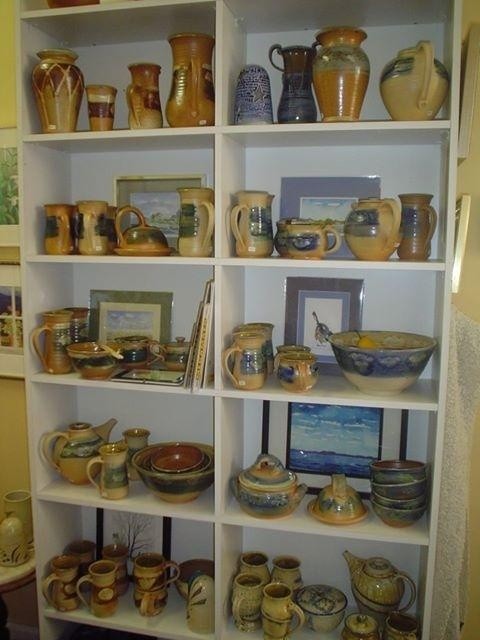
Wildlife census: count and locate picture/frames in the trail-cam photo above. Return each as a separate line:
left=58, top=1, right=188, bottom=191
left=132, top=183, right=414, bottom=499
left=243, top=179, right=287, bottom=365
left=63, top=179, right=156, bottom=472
left=457, top=19, right=480, bottom=166
left=89, top=289, right=174, bottom=347
left=449, top=194, right=472, bottom=295
left=284, top=276, right=363, bottom=377
left=281, top=178, right=381, bottom=261
left=96, top=508, right=172, bottom=582
left=262, top=399, right=408, bottom=502
left=0, top=128, right=21, bottom=250
left=0, top=263, right=25, bottom=382
left=111, top=174, right=205, bottom=250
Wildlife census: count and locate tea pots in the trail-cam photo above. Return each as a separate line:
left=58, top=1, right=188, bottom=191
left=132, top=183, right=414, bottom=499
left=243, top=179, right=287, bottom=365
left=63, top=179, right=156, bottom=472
left=39, top=416, right=118, bottom=484
left=342, top=550, right=420, bottom=622
left=229, top=451, right=310, bottom=521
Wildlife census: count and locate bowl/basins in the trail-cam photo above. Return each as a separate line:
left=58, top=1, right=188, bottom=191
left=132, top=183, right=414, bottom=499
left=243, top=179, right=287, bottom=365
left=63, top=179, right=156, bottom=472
left=326, top=329, right=438, bottom=396
left=65, top=341, right=124, bottom=381
left=131, top=440, right=215, bottom=504
left=369, top=458, right=429, bottom=528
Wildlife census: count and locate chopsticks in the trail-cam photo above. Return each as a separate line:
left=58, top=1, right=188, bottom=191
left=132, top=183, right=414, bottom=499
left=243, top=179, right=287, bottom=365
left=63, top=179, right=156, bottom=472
left=96, top=341, right=124, bottom=360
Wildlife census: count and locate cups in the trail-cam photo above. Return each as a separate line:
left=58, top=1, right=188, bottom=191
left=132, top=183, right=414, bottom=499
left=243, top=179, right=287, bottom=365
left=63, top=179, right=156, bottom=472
left=41, top=539, right=180, bottom=617
left=85, top=427, right=150, bottom=500
left=222, top=322, right=274, bottom=389
left=177, top=187, right=276, bottom=257
left=43, top=200, right=117, bottom=256
left=85, top=84, right=117, bottom=131
left=29, top=307, right=88, bottom=373
left=398, top=193, right=438, bottom=262
left=232, top=551, right=305, bottom=640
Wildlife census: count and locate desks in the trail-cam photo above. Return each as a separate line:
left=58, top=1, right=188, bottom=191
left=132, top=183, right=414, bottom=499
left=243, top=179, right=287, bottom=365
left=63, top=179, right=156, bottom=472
left=1, top=557, right=37, bottom=640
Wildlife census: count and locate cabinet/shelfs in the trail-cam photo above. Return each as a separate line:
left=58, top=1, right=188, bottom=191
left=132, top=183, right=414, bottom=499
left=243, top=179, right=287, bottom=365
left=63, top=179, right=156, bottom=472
left=14, top=0, right=461, bottom=639
